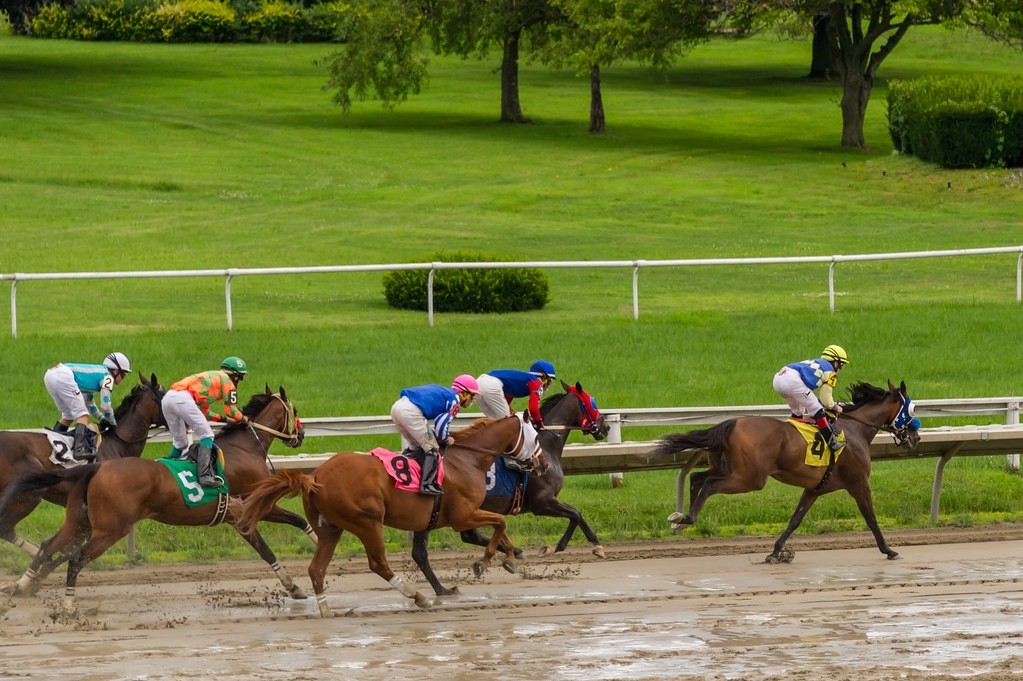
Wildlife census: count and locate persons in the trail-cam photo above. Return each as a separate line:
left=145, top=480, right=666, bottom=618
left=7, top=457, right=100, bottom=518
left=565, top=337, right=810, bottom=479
left=773, top=345, right=850, bottom=451
left=44, top=352, right=132, bottom=460
left=162, top=357, right=247, bottom=487
left=475, top=362, right=556, bottom=468
left=392, top=374, right=480, bottom=495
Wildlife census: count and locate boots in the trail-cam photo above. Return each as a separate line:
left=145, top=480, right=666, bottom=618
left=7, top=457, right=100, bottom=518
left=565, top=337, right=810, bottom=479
left=419, top=455, right=445, bottom=495
left=74, top=423, right=97, bottom=460
left=53, top=421, right=69, bottom=433
left=819, top=426, right=845, bottom=451
left=198, top=447, right=221, bottom=487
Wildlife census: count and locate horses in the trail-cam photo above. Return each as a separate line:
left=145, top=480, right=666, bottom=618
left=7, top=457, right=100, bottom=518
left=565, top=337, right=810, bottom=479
left=410, top=380, right=611, bottom=596
left=0, top=383, right=317, bottom=609
left=0, top=370, right=189, bottom=559
left=649, top=380, right=922, bottom=566
left=236, top=412, right=549, bottom=621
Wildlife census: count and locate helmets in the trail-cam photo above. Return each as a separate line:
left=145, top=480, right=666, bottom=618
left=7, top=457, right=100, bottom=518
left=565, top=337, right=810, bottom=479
left=103, top=352, right=132, bottom=372
left=821, top=345, right=849, bottom=364
left=220, top=356, right=247, bottom=375
left=529, top=360, right=557, bottom=378
left=451, top=374, right=482, bottom=395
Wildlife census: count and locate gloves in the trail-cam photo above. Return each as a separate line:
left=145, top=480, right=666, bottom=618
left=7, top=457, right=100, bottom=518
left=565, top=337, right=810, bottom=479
left=534, top=419, right=547, bottom=431
